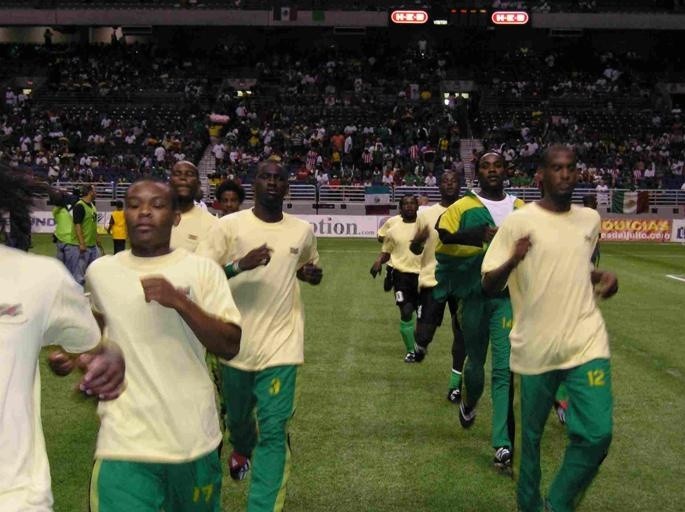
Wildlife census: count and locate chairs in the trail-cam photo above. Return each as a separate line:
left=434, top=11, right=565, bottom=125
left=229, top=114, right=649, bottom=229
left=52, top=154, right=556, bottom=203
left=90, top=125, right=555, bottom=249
left=61, top=101, right=199, bottom=131
left=310, top=86, right=388, bottom=130
left=476, top=100, right=666, bottom=150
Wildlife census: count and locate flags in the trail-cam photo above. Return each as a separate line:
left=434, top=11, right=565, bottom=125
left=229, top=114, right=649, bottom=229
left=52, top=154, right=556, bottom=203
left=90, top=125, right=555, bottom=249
left=364, top=185, right=389, bottom=217
left=612, top=192, right=649, bottom=214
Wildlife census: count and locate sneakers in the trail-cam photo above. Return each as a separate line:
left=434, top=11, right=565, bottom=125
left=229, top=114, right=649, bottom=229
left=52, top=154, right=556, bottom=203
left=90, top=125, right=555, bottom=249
left=384, top=264, right=394, bottom=291
left=448, top=388, right=461, bottom=403
left=555, top=399, right=567, bottom=424
left=229, top=452, right=249, bottom=481
left=494, top=447, right=512, bottom=468
left=415, top=343, right=426, bottom=362
left=405, top=352, right=415, bottom=363
left=459, top=401, right=476, bottom=428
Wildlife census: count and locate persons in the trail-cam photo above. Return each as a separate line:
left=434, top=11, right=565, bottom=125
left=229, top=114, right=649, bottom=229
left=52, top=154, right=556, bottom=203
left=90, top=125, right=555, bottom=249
left=166, top=161, right=246, bottom=255
left=431, top=149, right=526, bottom=472
left=371, top=170, right=464, bottom=404
left=49, top=184, right=126, bottom=284
left=47, top=179, right=242, bottom=512
left=193, top=161, right=322, bottom=511
left=1, top=28, right=685, bottom=202
left=480, top=140, right=618, bottom=511
left=0, top=163, right=128, bottom=512
